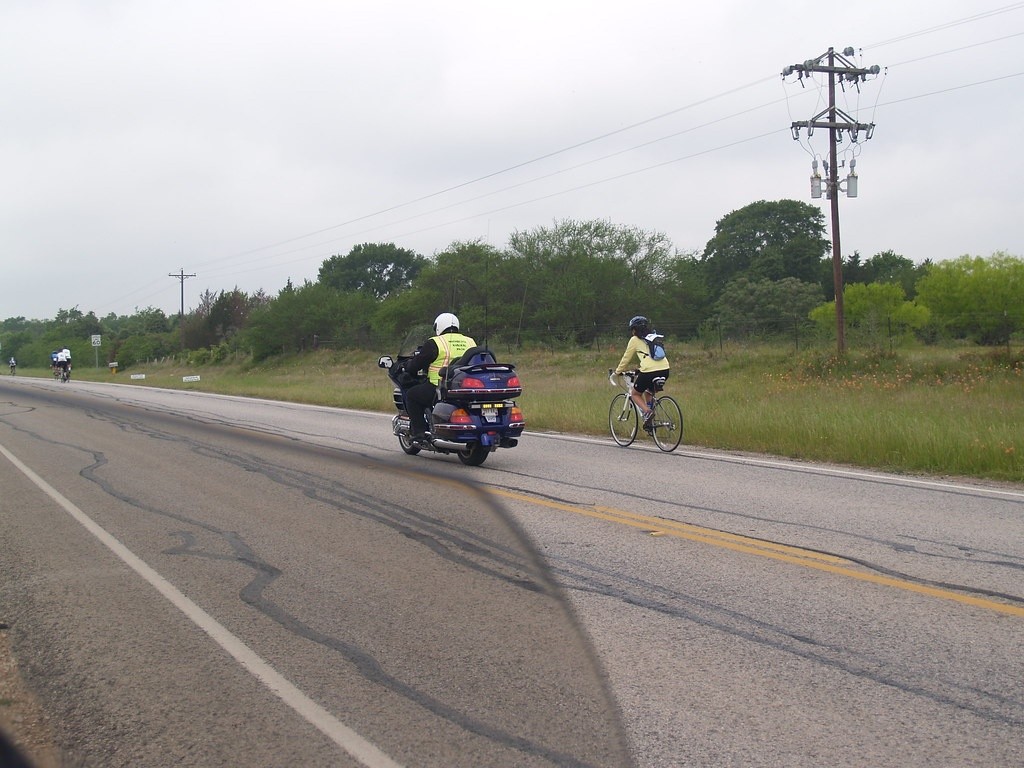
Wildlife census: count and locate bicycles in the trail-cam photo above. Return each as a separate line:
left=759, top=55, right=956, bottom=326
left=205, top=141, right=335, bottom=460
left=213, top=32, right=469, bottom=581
left=9, top=366, right=16, bottom=375
left=608, top=367, right=683, bottom=452
left=50, top=362, right=71, bottom=383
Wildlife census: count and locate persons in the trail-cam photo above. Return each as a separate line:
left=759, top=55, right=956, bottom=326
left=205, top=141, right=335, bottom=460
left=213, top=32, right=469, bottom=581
left=8, top=346, right=71, bottom=379
left=402, top=312, right=478, bottom=440
left=613, top=316, right=670, bottom=428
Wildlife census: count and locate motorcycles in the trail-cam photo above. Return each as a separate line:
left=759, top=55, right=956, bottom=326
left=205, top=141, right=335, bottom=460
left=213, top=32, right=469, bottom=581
left=378, top=343, right=526, bottom=466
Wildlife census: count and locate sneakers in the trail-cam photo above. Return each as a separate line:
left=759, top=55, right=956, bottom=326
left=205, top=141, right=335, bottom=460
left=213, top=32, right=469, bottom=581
left=642, top=410, right=654, bottom=427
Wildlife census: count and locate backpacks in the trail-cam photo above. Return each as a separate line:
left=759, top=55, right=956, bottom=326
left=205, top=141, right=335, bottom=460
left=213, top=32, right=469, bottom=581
left=644, top=333, right=665, bottom=360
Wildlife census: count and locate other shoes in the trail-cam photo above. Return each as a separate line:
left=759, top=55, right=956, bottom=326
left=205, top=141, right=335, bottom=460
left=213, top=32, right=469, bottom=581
left=409, top=432, right=425, bottom=440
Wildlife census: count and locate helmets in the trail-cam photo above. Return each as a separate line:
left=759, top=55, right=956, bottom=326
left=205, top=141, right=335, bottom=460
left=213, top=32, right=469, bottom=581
left=432, top=313, right=460, bottom=336
left=629, top=316, right=647, bottom=327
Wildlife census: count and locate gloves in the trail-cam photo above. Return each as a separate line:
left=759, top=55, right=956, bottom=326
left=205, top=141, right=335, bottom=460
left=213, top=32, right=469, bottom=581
left=615, top=372, right=624, bottom=376
left=634, top=369, right=640, bottom=375
